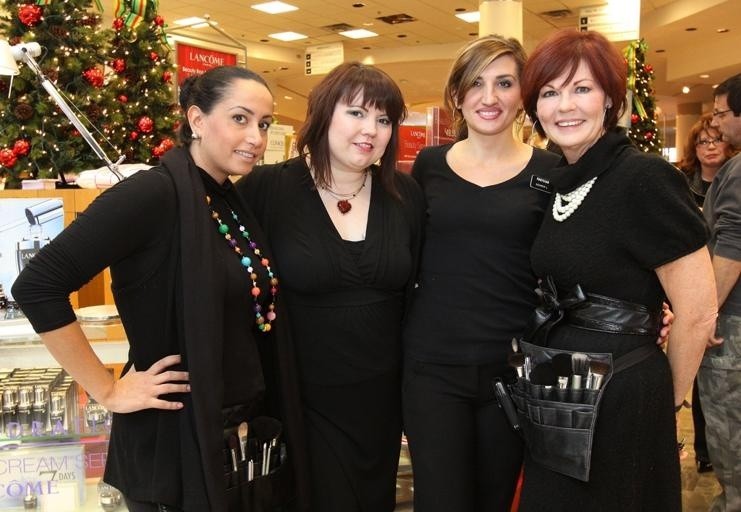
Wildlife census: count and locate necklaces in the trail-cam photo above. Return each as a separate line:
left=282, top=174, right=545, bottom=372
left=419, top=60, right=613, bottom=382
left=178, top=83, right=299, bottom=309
left=319, top=168, right=367, bottom=214
left=203, top=189, right=277, bottom=333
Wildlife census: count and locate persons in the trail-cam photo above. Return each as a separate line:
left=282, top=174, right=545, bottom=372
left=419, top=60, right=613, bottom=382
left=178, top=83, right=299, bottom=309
left=402, top=34, right=673, bottom=512
left=11, top=66, right=279, bottom=510
left=232, top=59, right=426, bottom=512
left=511, top=29, right=717, bottom=511
left=678, top=112, right=741, bottom=473
left=700, top=72, right=741, bottom=512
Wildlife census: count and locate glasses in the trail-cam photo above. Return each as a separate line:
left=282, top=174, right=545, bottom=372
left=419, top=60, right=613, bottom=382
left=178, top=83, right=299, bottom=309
left=696, top=139, right=724, bottom=147
left=712, top=110, right=733, bottom=120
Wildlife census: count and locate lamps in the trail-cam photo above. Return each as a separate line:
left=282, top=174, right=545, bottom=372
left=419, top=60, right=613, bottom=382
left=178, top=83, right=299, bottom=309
left=0, top=36, right=126, bottom=184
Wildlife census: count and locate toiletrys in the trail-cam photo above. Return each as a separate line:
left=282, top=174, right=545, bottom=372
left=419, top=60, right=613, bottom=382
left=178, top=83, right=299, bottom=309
left=0, top=363, right=125, bottom=512
